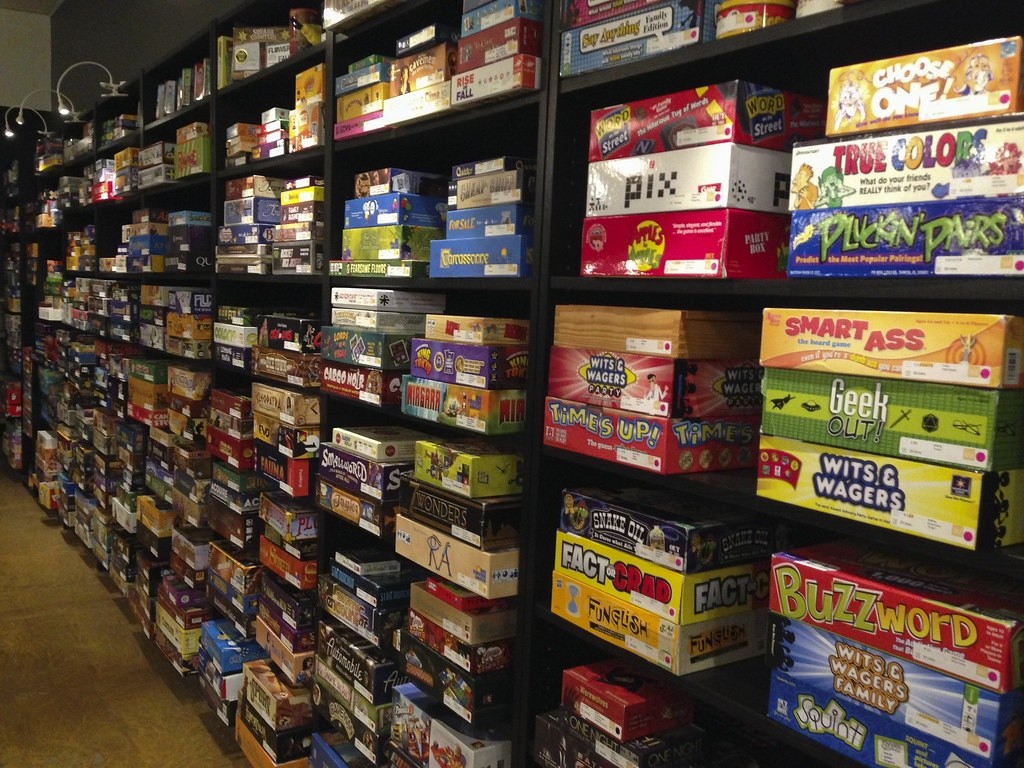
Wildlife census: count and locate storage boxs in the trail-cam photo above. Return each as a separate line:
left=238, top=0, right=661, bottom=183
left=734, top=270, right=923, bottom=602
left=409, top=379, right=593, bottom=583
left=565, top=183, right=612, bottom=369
left=0, top=0, right=1024, bottom=768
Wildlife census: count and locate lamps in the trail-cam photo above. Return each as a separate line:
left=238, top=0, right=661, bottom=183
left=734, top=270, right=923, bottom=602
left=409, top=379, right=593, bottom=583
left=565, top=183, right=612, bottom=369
left=15, top=87, right=89, bottom=125
left=56, top=61, right=129, bottom=117
left=4, top=105, right=55, bottom=139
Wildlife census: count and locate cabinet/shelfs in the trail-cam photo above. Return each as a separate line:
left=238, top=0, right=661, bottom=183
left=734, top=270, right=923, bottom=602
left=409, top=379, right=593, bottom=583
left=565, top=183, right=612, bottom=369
left=519, top=0, right=1024, bottom=768
left=0, top=0, right=555, bottom=768
